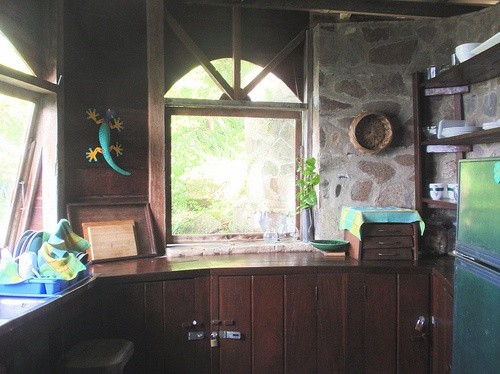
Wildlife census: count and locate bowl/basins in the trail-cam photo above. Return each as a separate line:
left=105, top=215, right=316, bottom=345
left=454, top=42, right=482, bottom=63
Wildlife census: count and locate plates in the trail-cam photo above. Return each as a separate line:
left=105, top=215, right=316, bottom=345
left=309, top=240, right=349, bottom=251
left=440, top=119, right=500, bottom=138
left=13, top=230, right=49, bottom=265
left=471, top=31, right=500, bottom=54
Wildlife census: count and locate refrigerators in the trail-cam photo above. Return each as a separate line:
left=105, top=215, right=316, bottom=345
left=450, top=156, right=500, bottom=374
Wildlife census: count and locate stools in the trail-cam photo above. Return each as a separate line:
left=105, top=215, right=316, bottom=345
left=66, top=339, right=133, bottom=374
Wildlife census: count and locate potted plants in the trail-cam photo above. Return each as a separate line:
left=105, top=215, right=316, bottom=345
left=290, top=158, right=318, bottom=242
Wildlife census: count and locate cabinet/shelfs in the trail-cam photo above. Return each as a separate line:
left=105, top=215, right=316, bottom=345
left=0, top=47, right=500, bottom=374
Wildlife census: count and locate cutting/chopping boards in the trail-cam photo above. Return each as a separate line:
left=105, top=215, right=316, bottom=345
left=82, top=220, right=138, bottom=262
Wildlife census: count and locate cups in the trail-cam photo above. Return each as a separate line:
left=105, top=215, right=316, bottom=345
left=447, top=184, right=458, bottom=199
left=429, top=183, right=444, bottom=201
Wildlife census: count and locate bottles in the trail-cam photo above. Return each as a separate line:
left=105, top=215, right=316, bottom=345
left=425, top=221, right=447, bottom=256
left=447, top=222, right=456, bottom=256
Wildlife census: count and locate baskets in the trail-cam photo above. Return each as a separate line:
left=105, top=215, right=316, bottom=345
left=348, top=111, right=393, bottom=155
